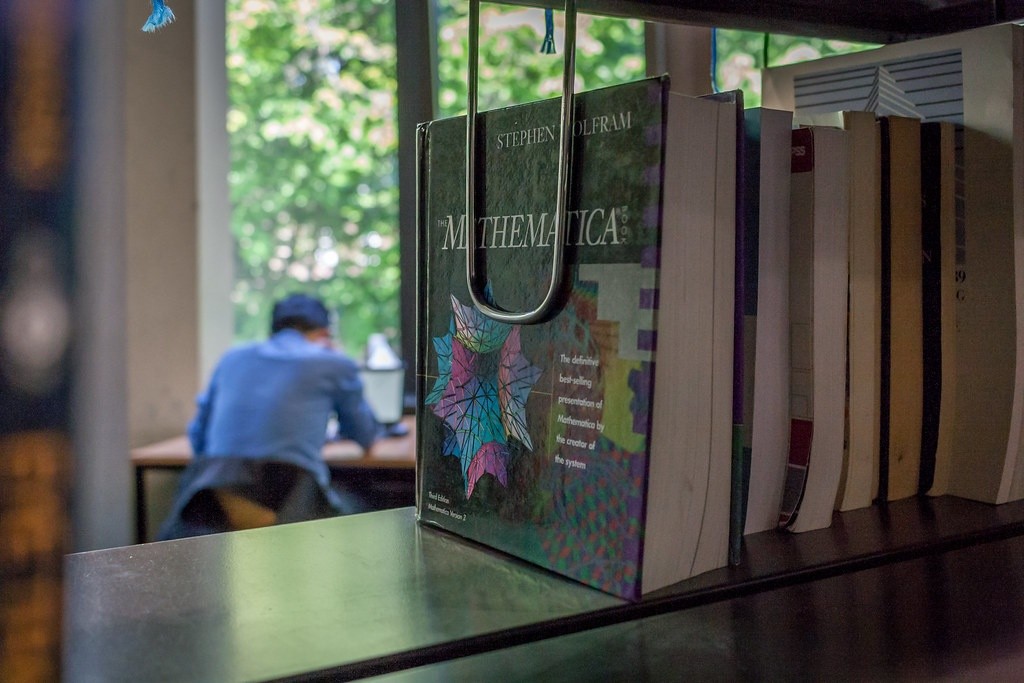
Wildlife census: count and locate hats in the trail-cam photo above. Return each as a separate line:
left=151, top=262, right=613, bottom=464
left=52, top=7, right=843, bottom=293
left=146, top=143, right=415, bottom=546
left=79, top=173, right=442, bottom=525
left=272, top=294, right=328, bottom=328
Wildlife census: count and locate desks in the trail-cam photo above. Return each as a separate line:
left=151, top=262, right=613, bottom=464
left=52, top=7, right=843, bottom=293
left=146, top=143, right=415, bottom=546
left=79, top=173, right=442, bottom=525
left=65, top=494, right=1024, bottom=683
left=132, top=414, right=416, bottom=545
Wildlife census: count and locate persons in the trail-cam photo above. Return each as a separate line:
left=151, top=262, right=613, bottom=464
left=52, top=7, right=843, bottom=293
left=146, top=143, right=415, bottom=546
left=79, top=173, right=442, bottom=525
left=189, top=294, right=380, bottom=521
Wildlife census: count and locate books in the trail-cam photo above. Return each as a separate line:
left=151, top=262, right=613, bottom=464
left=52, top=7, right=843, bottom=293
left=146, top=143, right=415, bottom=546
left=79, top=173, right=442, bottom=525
left=415, top=21, right=1023, bottom=607
